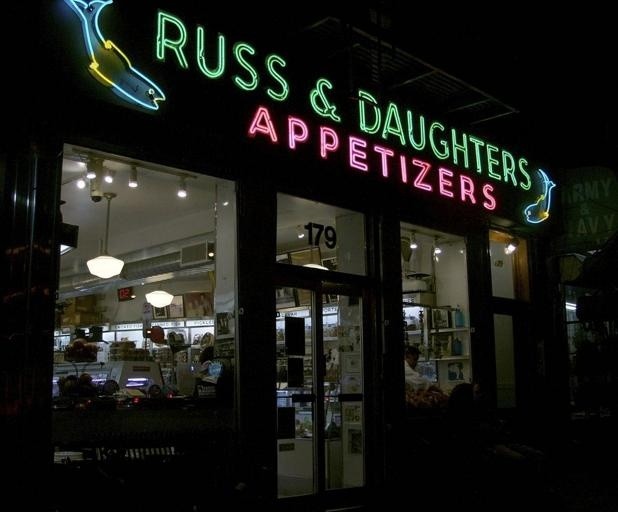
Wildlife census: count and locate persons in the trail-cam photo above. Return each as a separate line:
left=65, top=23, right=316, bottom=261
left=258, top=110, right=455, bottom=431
left=187, top=293, right=212, bottom=316
left=471, top=376, right=531, bottom=463
left=405, top=345, right=444, bottom=393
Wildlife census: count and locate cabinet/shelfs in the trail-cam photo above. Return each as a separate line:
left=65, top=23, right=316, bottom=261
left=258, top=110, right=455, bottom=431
left=277, top=326, right=470, bottom=366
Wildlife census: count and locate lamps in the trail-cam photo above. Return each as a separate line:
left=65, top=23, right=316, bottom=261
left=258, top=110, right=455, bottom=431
left=59, top=200, right=78, bottom=255
left=74, top=148, right=197, bottom=198
left=145, top=282, right=174, bottom=308
left=506, top=237, right=520, bottom=254
left=400, top=226, right=445, bottom=254
left=86, top=193, right=125, bottom=278
left=295, top=224, right=305, bottom=237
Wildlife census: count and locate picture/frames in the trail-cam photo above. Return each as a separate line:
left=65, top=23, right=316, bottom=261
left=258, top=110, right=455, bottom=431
left=152, top=305, right=168, bottom=319
left=168, top=294, right=185, bottom=318
